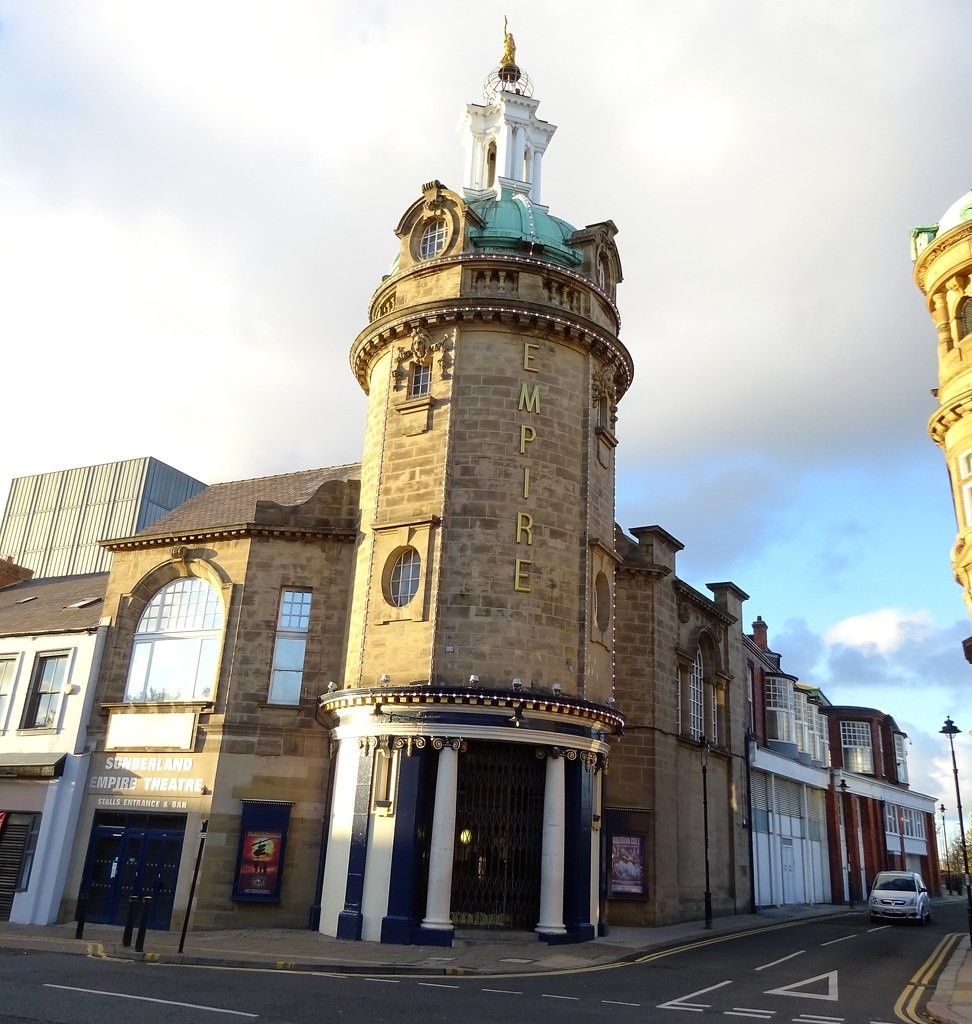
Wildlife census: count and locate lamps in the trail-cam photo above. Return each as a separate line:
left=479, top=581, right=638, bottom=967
left=551, top=684, right=562, bottom=698
left=327, top=681, right=338, bottom=692
left=468, top=675, right=480, bottom=689
left=512, top=678, right=523, bottom=692
left=380, top=674, right=391, bottom=687
left=605, top=697, right=616, bottom=710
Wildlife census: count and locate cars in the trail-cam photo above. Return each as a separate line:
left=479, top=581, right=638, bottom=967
left=868, top=870, right=932, bottom=926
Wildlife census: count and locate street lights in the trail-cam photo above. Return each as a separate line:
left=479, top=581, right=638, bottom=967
left=939, top=715, right=971, bottom=948
left=938, top=804, right=953, bottom=896
left=696, top=736, right=713, bottom=930
